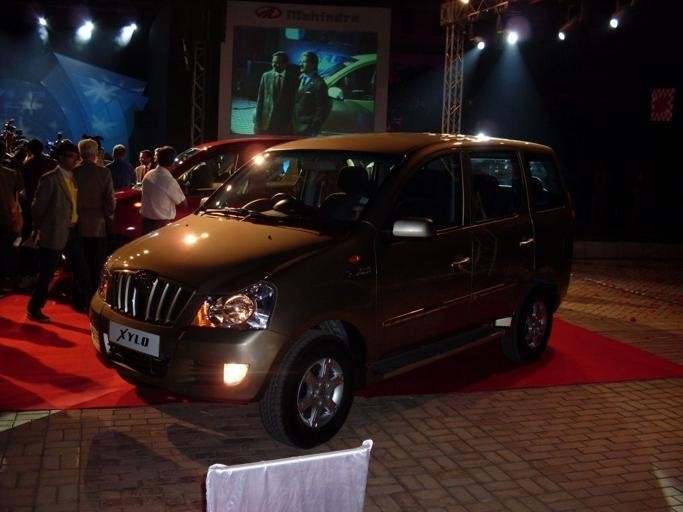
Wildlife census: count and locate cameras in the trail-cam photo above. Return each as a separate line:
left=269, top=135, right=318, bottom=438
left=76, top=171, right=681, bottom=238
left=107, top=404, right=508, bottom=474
left=4, top=118, right=16, bottom=132
left=54, top=132, right=63, bottom=145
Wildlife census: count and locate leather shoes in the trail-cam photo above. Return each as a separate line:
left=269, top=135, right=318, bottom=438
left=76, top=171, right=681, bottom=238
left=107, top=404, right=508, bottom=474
left=27, top=311, right=48, bottom=321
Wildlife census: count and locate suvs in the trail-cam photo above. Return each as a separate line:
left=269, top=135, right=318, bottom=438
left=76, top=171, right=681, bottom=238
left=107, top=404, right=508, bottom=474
left=113, top=134, right=307, bottom=249
left=320, top=54, right=377, bottom=133
left=89, top=133, right=578, bottom=449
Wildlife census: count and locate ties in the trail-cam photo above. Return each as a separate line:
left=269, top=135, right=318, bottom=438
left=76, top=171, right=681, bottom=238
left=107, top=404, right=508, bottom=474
left=276, top=76, right=281, bottom=97
left=298, top=75, right=306, bottom=94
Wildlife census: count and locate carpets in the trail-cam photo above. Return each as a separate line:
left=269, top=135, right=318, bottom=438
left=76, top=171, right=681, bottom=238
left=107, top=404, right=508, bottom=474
left=0, top=267, right=682, bottom=410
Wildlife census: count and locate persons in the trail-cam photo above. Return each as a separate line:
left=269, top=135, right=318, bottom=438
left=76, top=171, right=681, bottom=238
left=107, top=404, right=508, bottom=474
left=97, top=143, right=162, bottom=188
left=0, top=142, right=34, bottom=292
left=27, top=142, right=90, bottom=322
left=254, top=52, right=295, bottom=134
left=139, top=145, right=188, bottom=238
left=10, top=138, right=59, bottom=204
left=74, top=139, right=116, bottom=313
left=291, top=51, right=332, bottom=133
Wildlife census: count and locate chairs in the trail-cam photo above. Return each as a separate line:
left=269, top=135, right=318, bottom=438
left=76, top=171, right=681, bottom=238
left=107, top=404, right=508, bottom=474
left=319, top=165, right=376, bottom=221
left=205, top=439, right=375, bottom=512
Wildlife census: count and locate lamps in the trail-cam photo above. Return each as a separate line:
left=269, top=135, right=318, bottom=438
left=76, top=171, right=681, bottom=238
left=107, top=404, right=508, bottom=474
left=469, top=0, right=637, bottom=51
